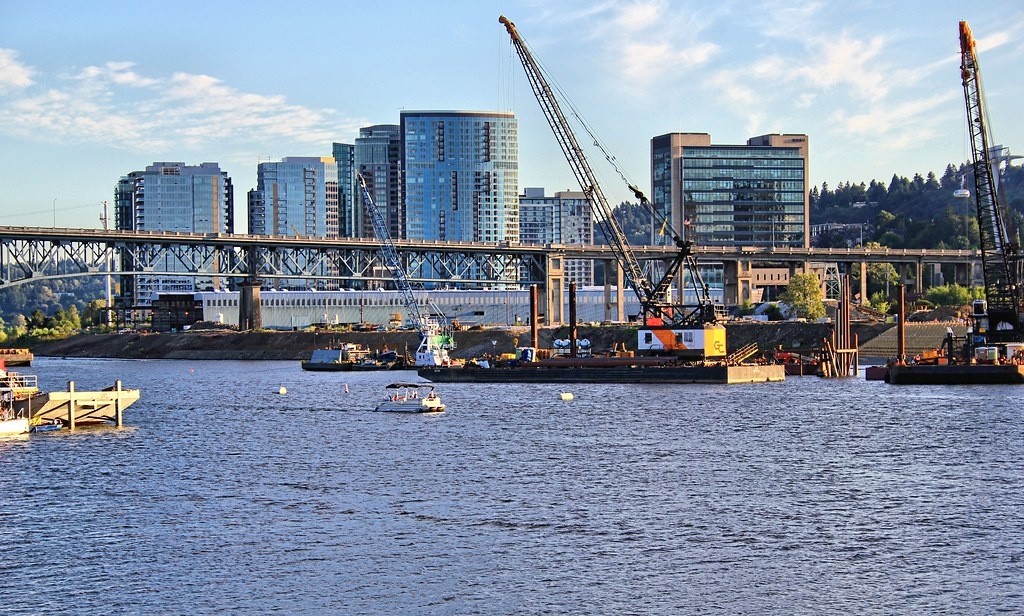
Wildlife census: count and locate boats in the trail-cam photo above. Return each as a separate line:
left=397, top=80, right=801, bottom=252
left=0, top=348, right=35, bottom=367
left=301, top=172, right=464, bottom=371
left=747, top=345, right=818, bottom=374
left=884, top=19, right=1023, bottom=382
left=0, top=369, right=48, bottom=435
left=30, top=417, right=64, bottom=432
left=374, top=381, right=445, bottom=413
left=414, top=14, right=785, bottom=385
